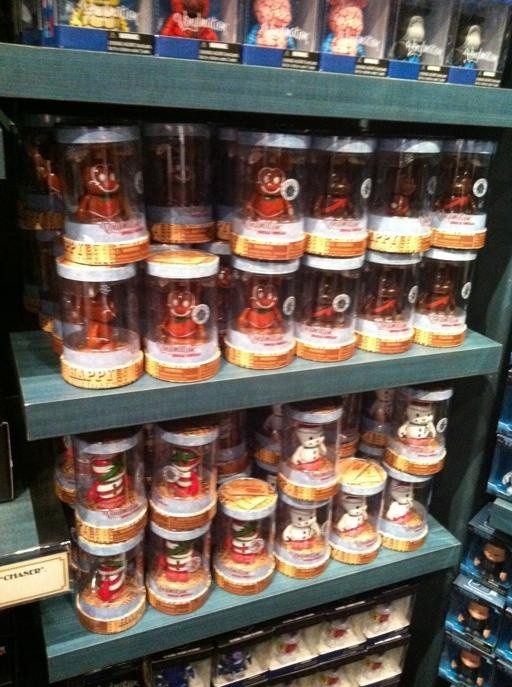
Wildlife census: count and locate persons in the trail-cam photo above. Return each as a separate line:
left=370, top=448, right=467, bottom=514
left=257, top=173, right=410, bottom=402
left=449, top=649, right=484, bottom=686
left=19, top=1, right=512, bottom=686
left=473, top=539, right=512, bottom=582
left=458, top=600, right=494, bottom=641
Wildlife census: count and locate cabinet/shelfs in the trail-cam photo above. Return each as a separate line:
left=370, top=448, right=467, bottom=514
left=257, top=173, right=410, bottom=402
left=3, top=17, right=512, bottom=685
